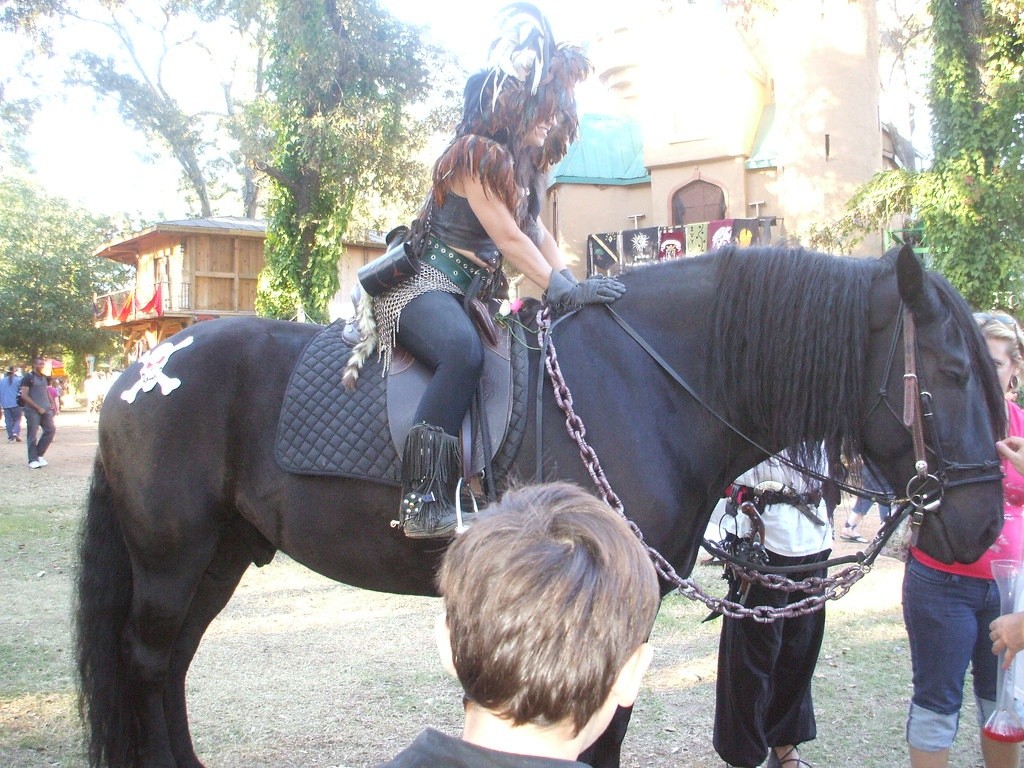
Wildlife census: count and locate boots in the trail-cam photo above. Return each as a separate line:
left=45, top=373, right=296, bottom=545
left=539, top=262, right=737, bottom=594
left=398, top=422, right=473, bottom=538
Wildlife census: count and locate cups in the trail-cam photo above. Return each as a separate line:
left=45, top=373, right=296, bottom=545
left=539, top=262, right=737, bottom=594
left=358, top=243, right=419, bottom=297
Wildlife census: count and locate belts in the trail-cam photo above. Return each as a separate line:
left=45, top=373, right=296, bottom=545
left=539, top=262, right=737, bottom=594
left=724, top=483, right=822, bottom=514
left=418, top=233, right=494, bottom=297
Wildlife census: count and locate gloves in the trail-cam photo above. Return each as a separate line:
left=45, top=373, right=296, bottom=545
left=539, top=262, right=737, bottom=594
left=542, top=268, right=627, bottom=311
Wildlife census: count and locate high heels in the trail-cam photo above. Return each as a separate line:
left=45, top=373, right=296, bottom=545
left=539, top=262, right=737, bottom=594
left=727, top=762, right=750, bottom=768
left=767, top=743, right=814, bottom=768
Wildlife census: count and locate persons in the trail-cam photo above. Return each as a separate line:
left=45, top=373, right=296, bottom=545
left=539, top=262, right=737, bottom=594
left=712, top=433, right=847, bottom=768
left=0, top=358, right=63, bottom=443
left=901, top=310, right=1024, bottom=768
left=20, top=357, right=56, bottom=469
left=840, top=458, right=894, bottom=544
left=371, top=42, right=624, bottom=537
left=382, top=482, right=661, bottom=768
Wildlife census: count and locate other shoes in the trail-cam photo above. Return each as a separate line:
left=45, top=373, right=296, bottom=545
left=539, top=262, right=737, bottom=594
left=8, top=434, right=21, bottom=443
left=29, top=455, right=47, bottom=468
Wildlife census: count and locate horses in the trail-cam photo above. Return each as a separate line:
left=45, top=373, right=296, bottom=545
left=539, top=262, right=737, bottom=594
left=73, top=242, right=1006, bottom=768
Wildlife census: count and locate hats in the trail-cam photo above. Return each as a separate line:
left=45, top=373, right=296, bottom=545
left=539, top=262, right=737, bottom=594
left=5, top=367, right=17, bottom=373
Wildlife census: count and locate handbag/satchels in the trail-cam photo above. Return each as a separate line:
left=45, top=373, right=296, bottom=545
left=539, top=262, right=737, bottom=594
left=16, top=373, right=34, bottom=406
